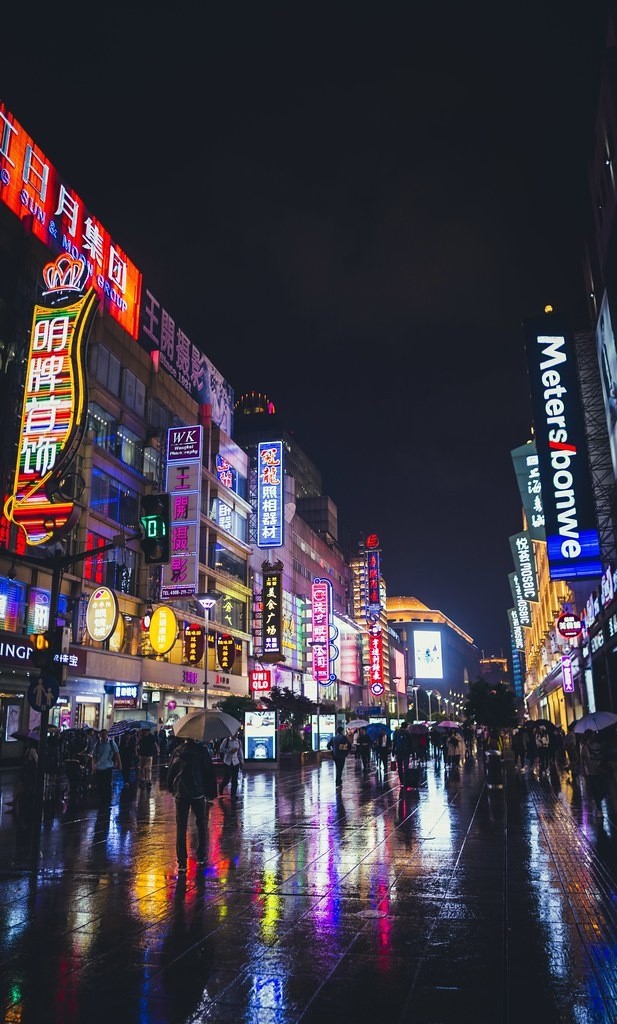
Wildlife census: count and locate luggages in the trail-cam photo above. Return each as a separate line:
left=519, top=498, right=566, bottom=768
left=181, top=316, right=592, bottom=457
left=405, top=752, right=420, bottom=788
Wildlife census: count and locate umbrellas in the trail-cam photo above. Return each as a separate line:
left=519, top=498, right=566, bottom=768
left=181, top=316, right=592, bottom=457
left=160, top=717, right=177, bottom=730
left=125, top=720, right=155, bottom=730
left=108, top=719, right=136, bottom=736
left=345, top=720, right=369, bottom=728
left=521, top=719, right=536, bottom=728
left=28, top=724, right=60, bottom=738
left=437, top=721, right=459, bottom=728
left=366, top=723, right=393, bottom=742
left=572, top=712, right=616, bottom=734
left=409, top=724, right=428, bottom=733
left=173, top=708, right=241, bottom=742
left=82, top=727, right=99, bottom=733
left=9, top=728, right=35, bottom=740
left=303, top=725, right=311, bottom=733
left=536, top=718, right=559, bottom=733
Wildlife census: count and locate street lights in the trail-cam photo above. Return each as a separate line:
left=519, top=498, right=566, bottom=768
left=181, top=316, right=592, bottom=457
left=445, top=698, right=449, bottom=717
left=362, top=664, right=371, bottom=707
left=309, top=640, right=325, bottom=763
left=456, top=703, right=459, bottom=722
left=450, top=701, right=455, bottom=722
left=392, top=676, right=401, bottom=727
left=411, top=684, right=420, bottom=724
left=460, top=705, right=463, bottom=722
left=436, top=695, right=442, bottom=713
left=192, top=591, right=223, bottom=710
left=425, top=688, right=433, bottom=722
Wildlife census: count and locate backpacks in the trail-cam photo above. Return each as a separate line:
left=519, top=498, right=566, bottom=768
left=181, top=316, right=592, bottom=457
left=167, top=742, right=203, bottom=792
left=397, top=730, right=412, bottom=751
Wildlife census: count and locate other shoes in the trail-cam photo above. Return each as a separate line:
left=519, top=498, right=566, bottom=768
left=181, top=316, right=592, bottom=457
left=178, top=864, right=187, bottom=871
left=232, top=795, right=238, bottom=799
left=597, top=810, right=602, bottom=818
left=336, top=785, right=343, bottom=789
left=197, top=856, right=209, bottom=864
left=219, top=784, right=223, bottom=794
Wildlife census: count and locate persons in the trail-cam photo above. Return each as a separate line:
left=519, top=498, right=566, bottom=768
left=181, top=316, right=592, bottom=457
left=8, top=709, right=19, bottom=736
left=510, top=725, right=616, bottom=817
left=219, top=729, right=245, bottom=801
left=356, top=722, right=506, bottom=789
left=166, top=742, right=217, bottom=871
left=25, top=729, right=174, bottom=806
left=327, top=727, right=352, bottom=790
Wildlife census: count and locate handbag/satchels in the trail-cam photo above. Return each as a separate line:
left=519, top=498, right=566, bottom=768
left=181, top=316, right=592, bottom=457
left=391, top=757, right=396, bottom=771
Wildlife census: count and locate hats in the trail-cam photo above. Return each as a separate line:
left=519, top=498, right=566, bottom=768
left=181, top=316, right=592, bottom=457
left=540, top=725, right=547, bottom=731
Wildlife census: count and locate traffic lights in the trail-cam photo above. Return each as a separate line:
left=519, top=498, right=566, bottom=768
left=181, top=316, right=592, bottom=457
left=138, top=491, right=172, bottom=565
left=27, top=629, right=54, bottom=671
